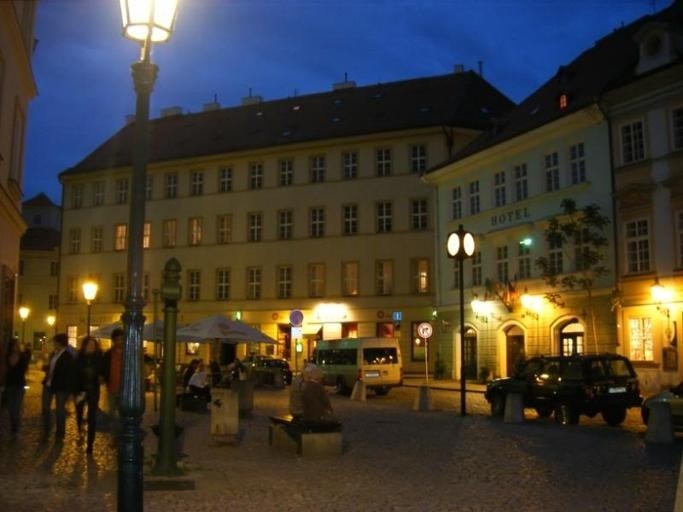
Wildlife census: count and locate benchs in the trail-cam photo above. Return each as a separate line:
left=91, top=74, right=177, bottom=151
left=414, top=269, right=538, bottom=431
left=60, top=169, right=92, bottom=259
left=267, top=414, right=344, bottom=457
left=180, top=390, right=209, bottom=414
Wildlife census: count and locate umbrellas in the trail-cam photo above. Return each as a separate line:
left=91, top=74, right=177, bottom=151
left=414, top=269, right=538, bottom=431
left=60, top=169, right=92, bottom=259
left=77, top=315, right=280, bottom=357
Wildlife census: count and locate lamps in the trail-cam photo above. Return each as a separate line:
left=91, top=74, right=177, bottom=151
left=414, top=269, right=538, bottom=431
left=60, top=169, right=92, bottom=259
left=650, top=277, right=665, bottom=301
left=519, top=294, right=542, bottom=320
left=471, top=299, right=497, bottom=323
left=432, top=307, right=438, bottom=319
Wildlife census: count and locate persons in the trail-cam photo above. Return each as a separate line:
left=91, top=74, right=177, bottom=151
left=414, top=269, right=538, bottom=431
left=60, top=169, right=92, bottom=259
left=288, top=377, right=305, bottom=418
left=301, top=363, right=344, bottom=426
left=102, top=328, right=123, bottom=447
left=41, top=334, right=75, bottom=437
left=0, top=330, right=32, bottom=441
left=143, top=354, right=156, bottom=377
left=181, top=352, right=254, bottom=403
left=71, top=337, right=110, bottom=454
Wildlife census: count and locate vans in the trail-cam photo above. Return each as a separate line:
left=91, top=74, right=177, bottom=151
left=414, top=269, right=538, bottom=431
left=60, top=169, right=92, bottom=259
left=303, top=336, right=402, bottom=397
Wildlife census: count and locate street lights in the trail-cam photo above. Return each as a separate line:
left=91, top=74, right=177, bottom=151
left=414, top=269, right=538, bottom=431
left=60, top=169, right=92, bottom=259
left=81, top=281, right=98, bottom=337
left=445, top=223, right=476, bottom=414
left=116, top=0, right=179, bottom=511
left=17, top=307, right=29, bottom=345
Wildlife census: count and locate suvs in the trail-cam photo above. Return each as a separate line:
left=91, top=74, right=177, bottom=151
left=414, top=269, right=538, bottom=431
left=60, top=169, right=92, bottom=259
left=484, top=351, right=644, bottom=426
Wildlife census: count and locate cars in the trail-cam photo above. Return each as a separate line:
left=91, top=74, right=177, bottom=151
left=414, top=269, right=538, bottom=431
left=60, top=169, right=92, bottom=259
left=247, top=358, right=292, bottom=384
left=641, top=381, right=683, bottom=431
left=227, top=355, right=273, bottom=369
left=142, top=355, right=156, bottom=389
